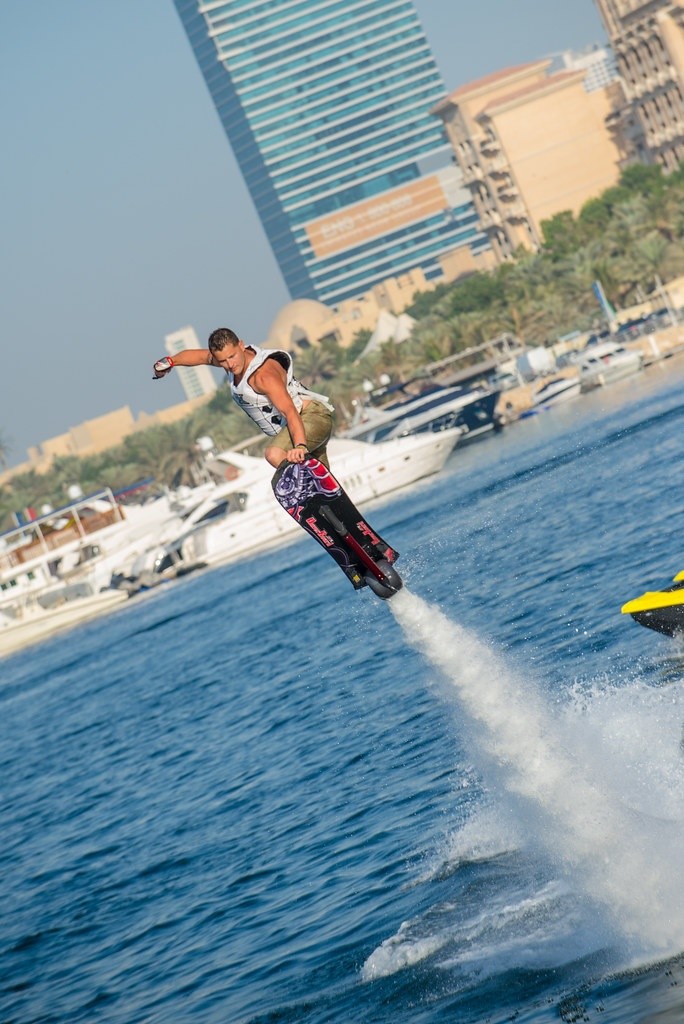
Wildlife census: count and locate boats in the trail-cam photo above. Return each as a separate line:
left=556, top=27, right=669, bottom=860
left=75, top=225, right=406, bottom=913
left=575, top=340, right=644, bottom=395
left=94, top=425, right=463, bottom=597
left=342, top=378, right=504, bottom=447
left=528, top=375, right=579, bottom=410
left=617, top=569, right=684, bottom=639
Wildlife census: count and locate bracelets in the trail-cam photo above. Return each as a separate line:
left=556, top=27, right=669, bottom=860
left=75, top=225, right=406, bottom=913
left=294, top=444, right=310, bottom=454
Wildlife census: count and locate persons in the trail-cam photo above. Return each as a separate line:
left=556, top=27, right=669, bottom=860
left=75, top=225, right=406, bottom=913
left=152, top=329, right=335, bottom=472
